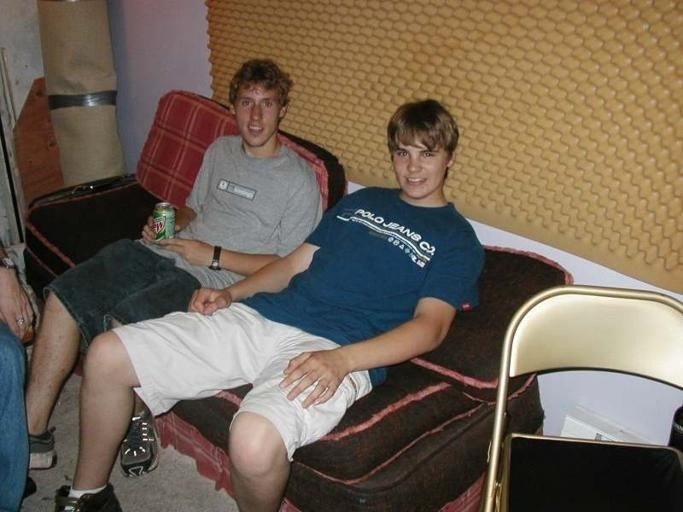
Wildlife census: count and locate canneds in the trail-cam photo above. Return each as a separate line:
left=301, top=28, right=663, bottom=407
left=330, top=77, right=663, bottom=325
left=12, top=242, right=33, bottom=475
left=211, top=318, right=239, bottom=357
left=152, top=201, right=176, bottom=242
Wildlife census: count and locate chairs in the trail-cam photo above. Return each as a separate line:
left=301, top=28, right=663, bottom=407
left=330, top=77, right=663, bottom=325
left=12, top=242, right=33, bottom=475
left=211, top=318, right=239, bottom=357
left=480, top=286, right=682, bottom=511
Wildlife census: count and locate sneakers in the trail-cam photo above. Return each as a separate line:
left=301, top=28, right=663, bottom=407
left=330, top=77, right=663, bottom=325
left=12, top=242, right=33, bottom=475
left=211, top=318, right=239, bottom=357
left=28, top=425, right=57, bottom=470
left=54, top=482, right=123, bottom=512
left=120, top=409, right=160, bottom=478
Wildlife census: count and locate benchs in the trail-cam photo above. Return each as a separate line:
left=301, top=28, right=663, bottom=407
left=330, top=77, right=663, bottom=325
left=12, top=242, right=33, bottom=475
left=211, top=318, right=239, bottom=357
left=15, top=88, right=570, bottom=511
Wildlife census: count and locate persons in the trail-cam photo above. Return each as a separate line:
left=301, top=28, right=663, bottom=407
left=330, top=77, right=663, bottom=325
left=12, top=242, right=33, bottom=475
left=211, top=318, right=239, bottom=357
left=26, top=60, right=322, bottom=477
left=0, top=244, right=35, bottom=512
left=53, top=100, right=486, bottom=512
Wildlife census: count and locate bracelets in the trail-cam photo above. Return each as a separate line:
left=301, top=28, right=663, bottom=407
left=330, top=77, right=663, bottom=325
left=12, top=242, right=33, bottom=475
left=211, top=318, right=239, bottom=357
left=209, top=246, right=223, bottom=271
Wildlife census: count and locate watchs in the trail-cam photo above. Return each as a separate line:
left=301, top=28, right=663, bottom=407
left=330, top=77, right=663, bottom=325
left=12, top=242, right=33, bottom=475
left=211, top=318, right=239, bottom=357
left=0, top=257, right=15, bottom=269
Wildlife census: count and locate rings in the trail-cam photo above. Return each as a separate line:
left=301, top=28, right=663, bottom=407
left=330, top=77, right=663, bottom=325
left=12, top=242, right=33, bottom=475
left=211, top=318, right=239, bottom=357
left=16, top=317, right=25, bottom=325
